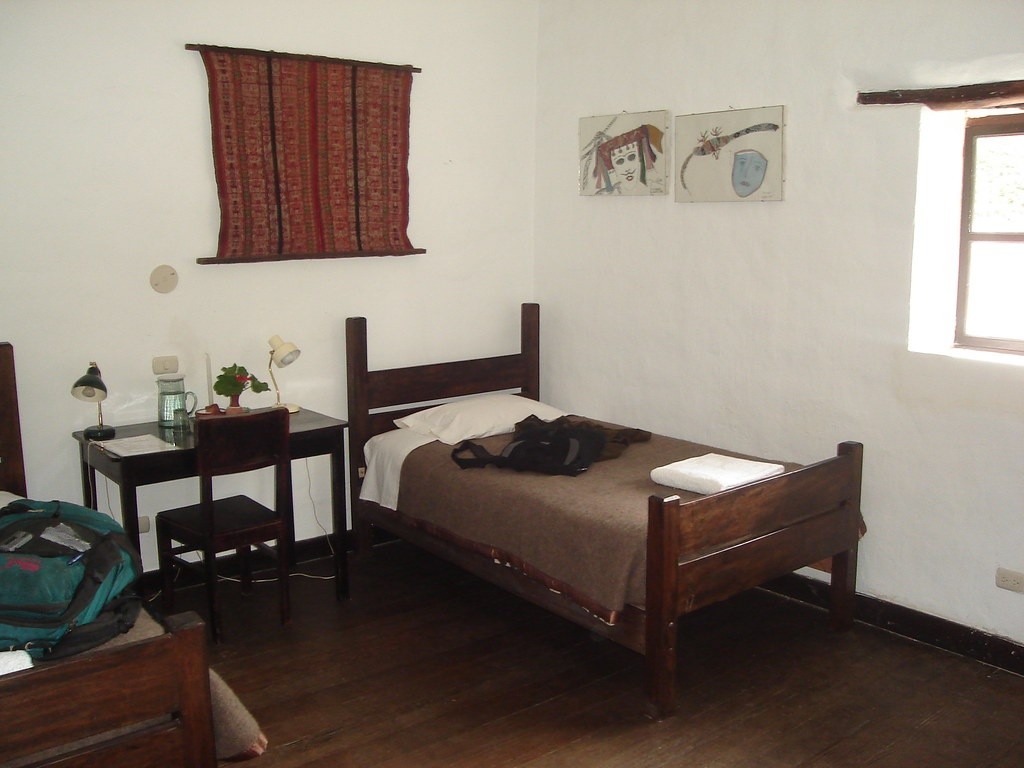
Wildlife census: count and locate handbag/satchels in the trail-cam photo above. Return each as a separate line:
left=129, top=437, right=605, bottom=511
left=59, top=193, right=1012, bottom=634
left=451, top=428, right=604, bottom=478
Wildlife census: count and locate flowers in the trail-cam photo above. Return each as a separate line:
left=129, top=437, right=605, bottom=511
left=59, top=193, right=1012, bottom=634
left=212, top=363, right=271, bottom=397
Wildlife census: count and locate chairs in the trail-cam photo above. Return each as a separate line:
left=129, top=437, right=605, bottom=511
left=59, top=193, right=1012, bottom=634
left=155, top=407, right=291, bottom=639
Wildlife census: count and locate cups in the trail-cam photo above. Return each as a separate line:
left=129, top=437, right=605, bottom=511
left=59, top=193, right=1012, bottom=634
left=173, top=409, right=190, bottom=433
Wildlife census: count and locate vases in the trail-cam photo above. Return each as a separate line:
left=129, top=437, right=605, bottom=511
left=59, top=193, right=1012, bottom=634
left=229, top=394, right=239, bottom=408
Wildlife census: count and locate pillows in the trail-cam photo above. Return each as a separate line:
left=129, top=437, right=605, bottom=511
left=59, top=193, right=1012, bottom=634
left=393, top=394, right=567, bottom=446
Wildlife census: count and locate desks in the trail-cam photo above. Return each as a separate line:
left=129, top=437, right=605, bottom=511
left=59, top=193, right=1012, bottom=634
left=71, top=403, right=350, bottom=601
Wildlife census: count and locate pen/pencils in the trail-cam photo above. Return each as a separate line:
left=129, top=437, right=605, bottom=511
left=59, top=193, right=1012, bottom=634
left=68, top=553, right=84, bottom=565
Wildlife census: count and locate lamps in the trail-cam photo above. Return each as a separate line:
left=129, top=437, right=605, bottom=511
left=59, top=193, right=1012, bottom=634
left=267, top=335, right=300, bottom=414
left=70, top=362, right=114, bottom=437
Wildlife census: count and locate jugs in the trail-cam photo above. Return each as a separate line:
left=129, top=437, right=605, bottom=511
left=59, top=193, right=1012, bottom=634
left=155, top=374, right=197, bottom=428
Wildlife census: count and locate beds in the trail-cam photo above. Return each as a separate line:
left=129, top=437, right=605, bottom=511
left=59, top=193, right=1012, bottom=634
left=345, top=303, right=867, bottom=722
left=0, top=341, right=270, bottom=768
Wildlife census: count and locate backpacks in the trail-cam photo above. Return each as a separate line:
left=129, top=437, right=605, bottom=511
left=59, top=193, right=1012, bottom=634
left=0, top=499, right=149, bottom=661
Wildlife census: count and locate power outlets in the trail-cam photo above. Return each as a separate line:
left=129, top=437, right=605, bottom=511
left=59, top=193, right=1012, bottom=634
left=358, top=467, right=365, bottom=478
left=996, top=567, right=1024, bottom=593
